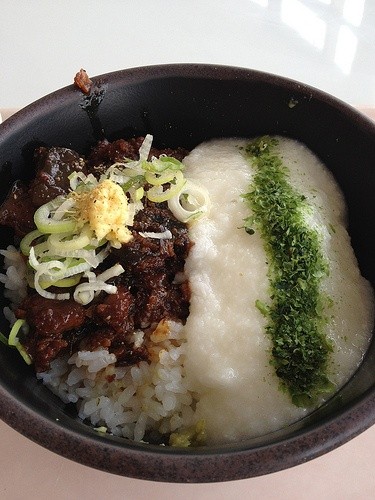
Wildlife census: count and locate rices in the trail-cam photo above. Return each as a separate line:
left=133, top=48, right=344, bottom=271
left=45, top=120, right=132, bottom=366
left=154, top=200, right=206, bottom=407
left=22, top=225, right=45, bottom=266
left=0, top=243, right=198, bottom=444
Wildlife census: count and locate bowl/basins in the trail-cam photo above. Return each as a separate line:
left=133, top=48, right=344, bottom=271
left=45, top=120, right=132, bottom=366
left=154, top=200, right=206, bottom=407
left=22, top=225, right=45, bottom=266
left=0, top=63, right=375, bottom=483
